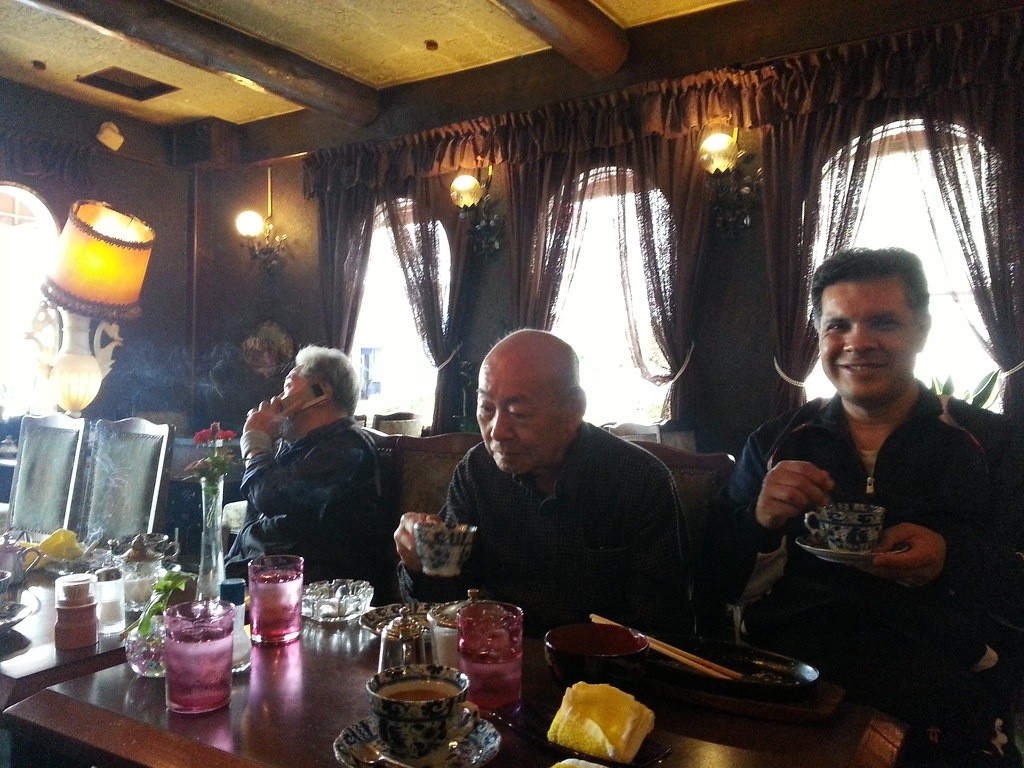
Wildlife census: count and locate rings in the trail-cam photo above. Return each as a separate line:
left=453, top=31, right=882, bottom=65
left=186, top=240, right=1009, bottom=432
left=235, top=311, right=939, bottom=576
left=282, top=415, right=290, bottom=421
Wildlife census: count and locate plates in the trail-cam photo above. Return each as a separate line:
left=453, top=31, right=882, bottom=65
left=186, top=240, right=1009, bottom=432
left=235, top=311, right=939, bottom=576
left=646, top=624, right=820, bottom=691
left=0, top=604, right=32, bottom=635
left=795, top=535, right=911, bottom=564
left=334, top=715, right=503, bottom=768
left=357, top=603, right=443, bottom=639
left=163, top=561, right=181, bottom=574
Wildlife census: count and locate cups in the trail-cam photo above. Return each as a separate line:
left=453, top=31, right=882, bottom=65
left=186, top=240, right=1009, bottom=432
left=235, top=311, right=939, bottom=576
left=248, top=556, right=304, bottom=643
left=456, top=601, right=522, bottom=714
left=365, top=664, right=469, bottom=757
left=164, top=600, right=235, bottom=713
left=426, top=588, right=509, bottom=668
left=118, top=534, right=181, bottom=558
left=804, top=504, right=886, bottom=551
left=0, top=570, right=12, bottom=615
left=414, top=520, right=478, bottom=576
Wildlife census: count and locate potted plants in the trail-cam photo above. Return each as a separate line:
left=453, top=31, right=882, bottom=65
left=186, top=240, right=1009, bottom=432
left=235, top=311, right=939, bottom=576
left=119, top=571, right=196, bottom=676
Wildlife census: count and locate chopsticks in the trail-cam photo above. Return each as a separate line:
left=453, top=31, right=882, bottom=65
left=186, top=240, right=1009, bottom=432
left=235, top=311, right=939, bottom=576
left=590, top=613, right=745, bottom=680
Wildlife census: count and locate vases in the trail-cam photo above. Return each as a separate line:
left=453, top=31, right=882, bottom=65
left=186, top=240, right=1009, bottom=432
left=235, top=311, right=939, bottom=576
left=198, top=476, right=226, bottom=598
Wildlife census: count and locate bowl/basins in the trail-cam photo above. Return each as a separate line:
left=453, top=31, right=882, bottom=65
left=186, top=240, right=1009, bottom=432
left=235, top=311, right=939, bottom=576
left=544, top=625, right=650, bottom=687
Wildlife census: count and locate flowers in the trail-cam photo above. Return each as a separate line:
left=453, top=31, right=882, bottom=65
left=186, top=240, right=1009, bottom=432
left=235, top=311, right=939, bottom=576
left=182, top=421, right=251, bottom=527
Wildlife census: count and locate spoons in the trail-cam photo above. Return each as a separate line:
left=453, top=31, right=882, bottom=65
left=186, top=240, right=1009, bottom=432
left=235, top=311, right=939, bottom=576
left=349, top=743, right=415, bottom=768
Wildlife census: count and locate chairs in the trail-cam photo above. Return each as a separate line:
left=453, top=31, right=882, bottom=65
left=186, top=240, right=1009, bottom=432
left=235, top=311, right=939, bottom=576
left=3, top=412, right=90, bottom=534
left=78, top=417, right=177, bottom=543
left=356, top=393, right=699, bottom=452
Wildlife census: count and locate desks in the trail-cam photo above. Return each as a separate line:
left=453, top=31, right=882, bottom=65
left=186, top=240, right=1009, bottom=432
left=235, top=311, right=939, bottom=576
left=0, top=582, right=912, bottom=768
left=170, top=434, right=244, bottom=560
left=0, top=534, right=209, bottom=710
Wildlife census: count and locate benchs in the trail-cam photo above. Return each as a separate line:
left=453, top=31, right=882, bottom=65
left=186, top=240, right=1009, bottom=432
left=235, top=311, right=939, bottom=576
left=374, top=432, right=736, bottom=578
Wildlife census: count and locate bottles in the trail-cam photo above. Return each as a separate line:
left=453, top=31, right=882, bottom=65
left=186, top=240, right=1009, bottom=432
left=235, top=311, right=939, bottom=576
left=218, top=577, right=251, bottom=671
left=378, top=607, right=425, bottom=675
left=92, top=568, right=127, bottom=634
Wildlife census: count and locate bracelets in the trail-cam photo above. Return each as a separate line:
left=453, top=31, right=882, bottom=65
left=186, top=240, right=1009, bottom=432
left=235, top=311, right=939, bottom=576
left=240, top=430, right=273, bottom=459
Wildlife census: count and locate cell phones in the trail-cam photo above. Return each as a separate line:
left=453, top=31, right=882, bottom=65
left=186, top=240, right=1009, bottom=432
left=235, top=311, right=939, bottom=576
left=278, top=382, right=327, bottom=416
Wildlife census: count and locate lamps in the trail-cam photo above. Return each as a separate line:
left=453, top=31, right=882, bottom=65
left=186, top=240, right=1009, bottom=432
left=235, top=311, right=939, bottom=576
left=234, top=210, right=287, bottom=282
left=449, top=174, right=506, bottom=267
left=698, top=132, right=764, bottom=239
left=25, top=199, right=157, bottom=416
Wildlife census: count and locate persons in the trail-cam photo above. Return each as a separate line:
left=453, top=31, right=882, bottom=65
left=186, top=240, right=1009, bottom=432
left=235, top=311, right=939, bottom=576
left=715, top=246, right=1023, bottom=768
left=392, top=328, right=712, bottom=740
left=224, top=321, right=390, bottom=607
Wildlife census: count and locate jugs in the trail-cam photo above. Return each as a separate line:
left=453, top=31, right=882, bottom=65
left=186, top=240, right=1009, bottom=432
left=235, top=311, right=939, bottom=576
left=112, top=535, right=169, bottom=611
left=0, top=531, right=40, bottom=584
